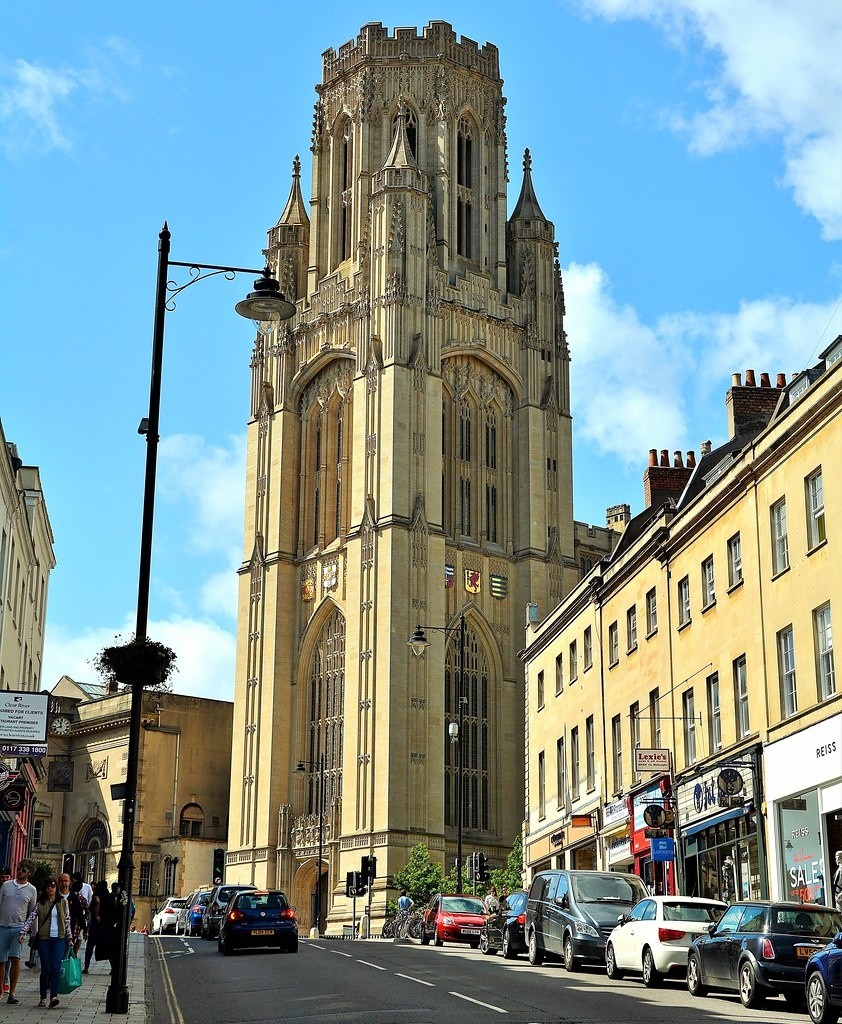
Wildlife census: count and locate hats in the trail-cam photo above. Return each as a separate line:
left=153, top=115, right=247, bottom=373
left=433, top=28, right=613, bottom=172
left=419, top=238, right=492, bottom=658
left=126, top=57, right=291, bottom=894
left=0, top=866, right=11, bottom=875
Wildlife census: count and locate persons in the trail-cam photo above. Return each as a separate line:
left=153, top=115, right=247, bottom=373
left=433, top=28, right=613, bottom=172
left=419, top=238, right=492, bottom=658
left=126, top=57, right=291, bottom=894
left=397, top=889, right=415, bottom=913
left=722, top=856, right=735, bottom=896
left=0, top=859, right=148, bottom=1008
left=485, top=886, right=499, bottom=915
left=832, top=851, right=842, bottom=910
left=498, top=886, right=511, bottom=903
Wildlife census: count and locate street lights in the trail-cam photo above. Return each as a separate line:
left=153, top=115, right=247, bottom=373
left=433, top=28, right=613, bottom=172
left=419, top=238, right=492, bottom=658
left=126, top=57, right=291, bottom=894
left=295, top=760, right=324, bottom=929
left=106, top=263, right=296, bottom=1014
left=448, top=697, right=468, bottom=894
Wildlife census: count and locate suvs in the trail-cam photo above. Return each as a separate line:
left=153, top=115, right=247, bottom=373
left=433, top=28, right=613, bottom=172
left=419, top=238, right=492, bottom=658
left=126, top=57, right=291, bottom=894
left=202, top=883, right=258, bottom=940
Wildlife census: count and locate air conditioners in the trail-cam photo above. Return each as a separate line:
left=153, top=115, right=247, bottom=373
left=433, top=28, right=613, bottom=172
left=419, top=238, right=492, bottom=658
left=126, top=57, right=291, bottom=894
left=85, top=632, right=182, bottom=715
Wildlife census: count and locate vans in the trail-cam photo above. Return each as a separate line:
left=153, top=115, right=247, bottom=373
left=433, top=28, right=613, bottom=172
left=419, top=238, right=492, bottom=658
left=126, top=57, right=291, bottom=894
left=523, top=869, right=650, bottom=972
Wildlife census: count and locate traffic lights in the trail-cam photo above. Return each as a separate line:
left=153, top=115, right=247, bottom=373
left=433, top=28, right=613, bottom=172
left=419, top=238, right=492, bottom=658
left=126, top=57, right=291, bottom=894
left=477, top=852, right=490, bottom=883
left=354, top=871, right=366, bottom=897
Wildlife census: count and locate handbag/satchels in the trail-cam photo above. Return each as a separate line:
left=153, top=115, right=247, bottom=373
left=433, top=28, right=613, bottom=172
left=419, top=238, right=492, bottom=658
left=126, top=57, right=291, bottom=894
left=27, top=932, right=39, bottom=948
left=57, top=946, right=83, bottom=994
left=94, top=943, right=108, bottom=961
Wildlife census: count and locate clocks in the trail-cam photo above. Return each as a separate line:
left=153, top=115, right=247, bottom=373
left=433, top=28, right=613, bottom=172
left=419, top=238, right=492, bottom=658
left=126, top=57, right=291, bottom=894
left=52, top=718, right=70, bottom=735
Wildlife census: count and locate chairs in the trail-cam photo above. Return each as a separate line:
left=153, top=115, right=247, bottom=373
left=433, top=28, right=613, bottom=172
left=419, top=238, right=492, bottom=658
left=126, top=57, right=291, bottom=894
left=268, top=896, right=280, bottom=907
left=221, top=893, right=228, bottom=900
left=240, top=897, right=251, bottom=909
left=795, top=913, right=813, bottom=932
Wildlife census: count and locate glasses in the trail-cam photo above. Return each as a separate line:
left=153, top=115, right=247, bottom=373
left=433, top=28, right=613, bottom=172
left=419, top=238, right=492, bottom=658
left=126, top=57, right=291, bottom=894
left=19, top=868, right=30, bottom=873
left=46, top=885, right=57, bottom=888
left=503, top=888, right=507, bottom=891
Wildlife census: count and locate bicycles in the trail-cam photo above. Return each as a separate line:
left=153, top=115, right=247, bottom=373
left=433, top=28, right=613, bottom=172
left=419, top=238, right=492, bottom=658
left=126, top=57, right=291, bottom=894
left=381, top=903, right=428, bottom=939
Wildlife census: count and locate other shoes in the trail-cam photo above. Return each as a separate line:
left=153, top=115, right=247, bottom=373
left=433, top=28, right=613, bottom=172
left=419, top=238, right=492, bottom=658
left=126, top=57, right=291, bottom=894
left=49, top=997, right=60, bottom=1008
left=38, top=998, right=47, bottom=1008
left=7, top=996, right=19, bottom=1003
left=25, top=961, right=33, bottom=968
left=82, top=968, right=88, bottom=974
left=2, top=983, right=10, bottom=993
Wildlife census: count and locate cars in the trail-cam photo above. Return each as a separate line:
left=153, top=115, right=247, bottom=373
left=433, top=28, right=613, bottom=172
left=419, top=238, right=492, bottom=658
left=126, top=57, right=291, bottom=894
left=218, top=889, right=299, bottom=954
left=804, top=927, right=842, bottom=1024
left=151, top=896, right=187, bottom=935
left=687, top=899, right=842, bottom=1010
left=605, top=894, right=730, bottom=988
left=175, top=890, right=213, bottom=935
left=421, top=893, right=490, bottom=948
left=479, top=890, right=530, bottom=958
